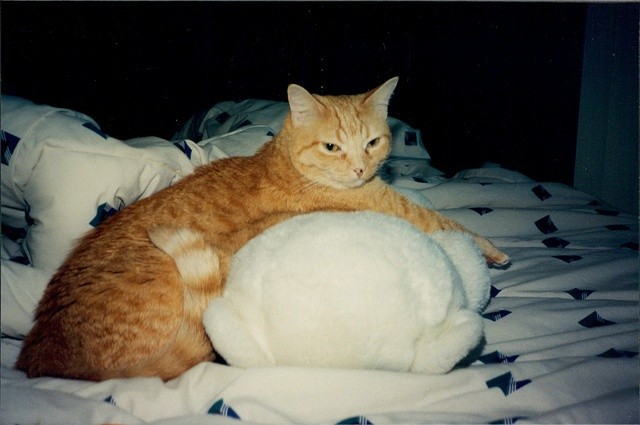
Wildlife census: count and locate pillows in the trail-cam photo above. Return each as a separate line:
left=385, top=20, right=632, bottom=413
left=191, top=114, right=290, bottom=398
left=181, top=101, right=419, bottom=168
left=200, top=99, right=431, bottom=180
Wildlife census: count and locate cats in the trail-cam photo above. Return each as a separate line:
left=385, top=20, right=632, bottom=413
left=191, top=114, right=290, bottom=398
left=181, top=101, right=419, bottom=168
left=13, top=75, right=511, bottom=383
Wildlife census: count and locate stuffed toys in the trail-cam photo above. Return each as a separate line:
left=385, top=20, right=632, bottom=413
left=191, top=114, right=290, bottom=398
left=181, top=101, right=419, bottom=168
left=201, top=186, right=493, bottom=377
